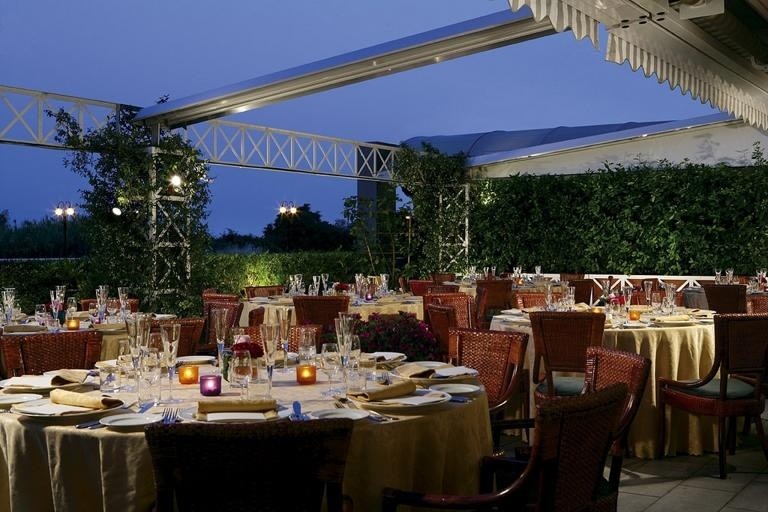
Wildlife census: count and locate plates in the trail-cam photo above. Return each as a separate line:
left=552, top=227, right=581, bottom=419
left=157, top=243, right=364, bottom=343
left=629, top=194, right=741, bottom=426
left=313, top=350, right=481, bottom=420
left=0, top=352, right=217, bottom=427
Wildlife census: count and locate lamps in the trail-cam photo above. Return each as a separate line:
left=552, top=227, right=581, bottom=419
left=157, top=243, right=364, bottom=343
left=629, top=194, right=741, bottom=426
left=110, top=204, right=127, bottom=215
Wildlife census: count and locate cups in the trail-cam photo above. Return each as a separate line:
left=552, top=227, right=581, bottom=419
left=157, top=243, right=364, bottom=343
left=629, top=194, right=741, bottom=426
left=1, top=284, right=131, bottom=329
left=463, top=262, right=542, bottom=283
left=713, top=266, right=767, bottom=293
left=287, top=269, right=390, bottom=295
left=538, top=277, right=676, bottom=327
left=123, top=304, right=364, bottom=407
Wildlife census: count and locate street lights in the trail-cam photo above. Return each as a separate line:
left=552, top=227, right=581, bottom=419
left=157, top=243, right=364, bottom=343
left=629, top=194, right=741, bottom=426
left=279, top=200, right=297, bottom=253
left=406, top=210, right=413, bottom=286
left=55, top=201, right=74, bottom=258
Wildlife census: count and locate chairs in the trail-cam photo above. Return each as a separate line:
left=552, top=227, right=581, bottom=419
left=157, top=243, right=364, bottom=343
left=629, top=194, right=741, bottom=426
left=0, top=266, right=768, bottom=512
